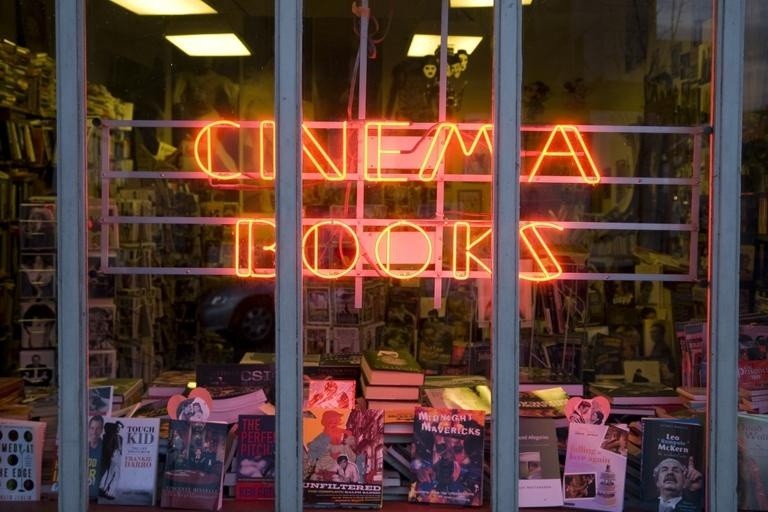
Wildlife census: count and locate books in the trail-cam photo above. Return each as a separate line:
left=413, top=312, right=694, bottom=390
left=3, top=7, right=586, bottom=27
left=1, top=37, right=766, bottom=512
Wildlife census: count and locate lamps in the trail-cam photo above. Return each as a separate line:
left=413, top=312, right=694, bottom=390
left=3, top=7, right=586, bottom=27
left=110, top=1, right=221, bottom=19
left=405, top=0, right=487, bottom=64
left=157, top=1, right=251, bottom=58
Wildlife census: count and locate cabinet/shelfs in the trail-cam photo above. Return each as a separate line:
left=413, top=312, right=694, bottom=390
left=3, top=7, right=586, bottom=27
left=0, top=39, right=137, bottom=382
